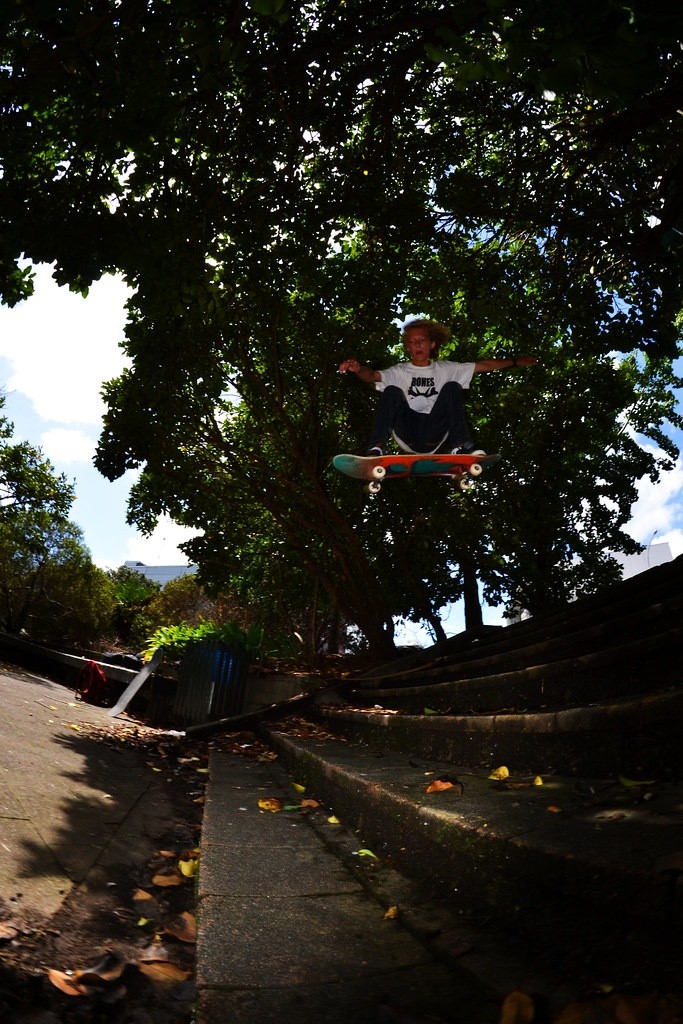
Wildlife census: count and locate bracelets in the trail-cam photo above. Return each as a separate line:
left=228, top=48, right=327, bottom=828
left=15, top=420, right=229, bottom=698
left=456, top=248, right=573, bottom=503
left=512, top=357, right=517, bottom=366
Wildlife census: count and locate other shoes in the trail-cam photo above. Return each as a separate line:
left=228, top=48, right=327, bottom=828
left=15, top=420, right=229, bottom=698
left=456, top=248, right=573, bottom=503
left=367, top=446, right=383, bottom=456
left=450, top=445, right=486, bottom=457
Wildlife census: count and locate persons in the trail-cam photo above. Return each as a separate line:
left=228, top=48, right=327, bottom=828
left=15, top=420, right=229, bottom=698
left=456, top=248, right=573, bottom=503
left=336, top=320, right=537, bottom=458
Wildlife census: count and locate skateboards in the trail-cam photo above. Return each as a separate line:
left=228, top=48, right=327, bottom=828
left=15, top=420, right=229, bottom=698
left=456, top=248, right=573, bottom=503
left=332, top=453, right=504, bottom=494
